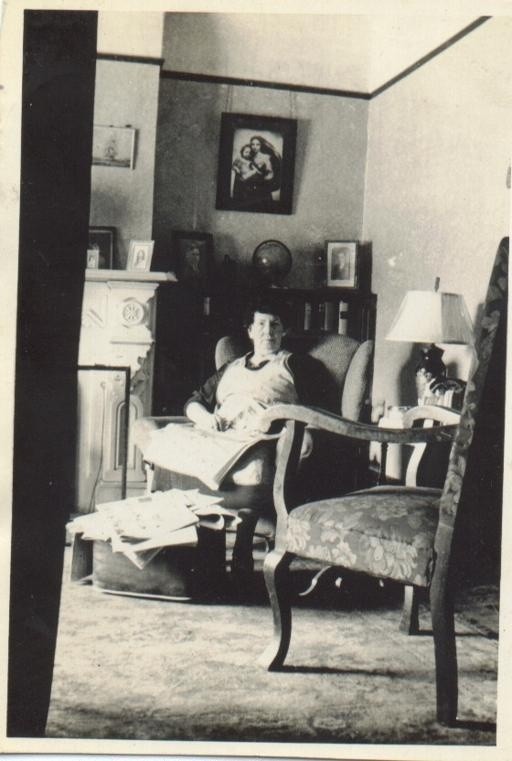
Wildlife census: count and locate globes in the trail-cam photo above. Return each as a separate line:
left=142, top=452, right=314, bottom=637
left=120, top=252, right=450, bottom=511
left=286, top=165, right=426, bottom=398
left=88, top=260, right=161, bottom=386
left=253, top=240, right=292, bottom=288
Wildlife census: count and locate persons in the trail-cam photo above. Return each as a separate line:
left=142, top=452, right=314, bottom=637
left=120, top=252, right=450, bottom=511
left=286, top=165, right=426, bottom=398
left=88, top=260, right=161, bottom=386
left=149, top=306, right=339, bottom=488
left=334, top=252, right=349, bottom=279
left=133, top=250, right=146, bottom=269
left=184, top=242, right=201, bottom=278
left=231, top=136, right=279, bottom=206
left=89, top=256, right=95, bottom=267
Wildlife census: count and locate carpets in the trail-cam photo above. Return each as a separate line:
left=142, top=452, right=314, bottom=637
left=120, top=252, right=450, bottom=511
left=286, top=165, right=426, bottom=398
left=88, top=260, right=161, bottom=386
left=43, top=513, right=498, bottom=746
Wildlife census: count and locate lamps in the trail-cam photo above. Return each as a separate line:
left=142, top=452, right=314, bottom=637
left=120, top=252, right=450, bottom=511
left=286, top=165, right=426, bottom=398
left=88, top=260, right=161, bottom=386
left=385, top=277, right=475, bottom=405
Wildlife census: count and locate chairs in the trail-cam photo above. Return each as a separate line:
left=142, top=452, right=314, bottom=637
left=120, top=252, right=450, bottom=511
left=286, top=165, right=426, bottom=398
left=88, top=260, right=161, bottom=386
left=256, top=236, right=509, bottom=727
left=131, top=327, right=374, bottom=592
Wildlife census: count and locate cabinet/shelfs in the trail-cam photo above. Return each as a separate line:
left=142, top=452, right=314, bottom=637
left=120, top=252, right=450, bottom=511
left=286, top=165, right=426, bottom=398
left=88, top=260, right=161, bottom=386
left=152, top=286, right=376, bottom=417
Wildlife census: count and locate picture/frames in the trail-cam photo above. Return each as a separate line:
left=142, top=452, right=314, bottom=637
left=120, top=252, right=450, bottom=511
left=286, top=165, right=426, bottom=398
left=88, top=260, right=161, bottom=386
left=86, top=225, right=116, bottom=270
left=216, top=112, right=298, bottom=215
left=323, top=239, right=360, bottom=290
left=92, top=124, right=136, bottom=170
left=172, top=230, right=213, bottom=278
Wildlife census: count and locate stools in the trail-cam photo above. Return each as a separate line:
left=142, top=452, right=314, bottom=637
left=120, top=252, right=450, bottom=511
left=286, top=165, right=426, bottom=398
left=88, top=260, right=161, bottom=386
left=93, top=514, right=226, bottom=604
